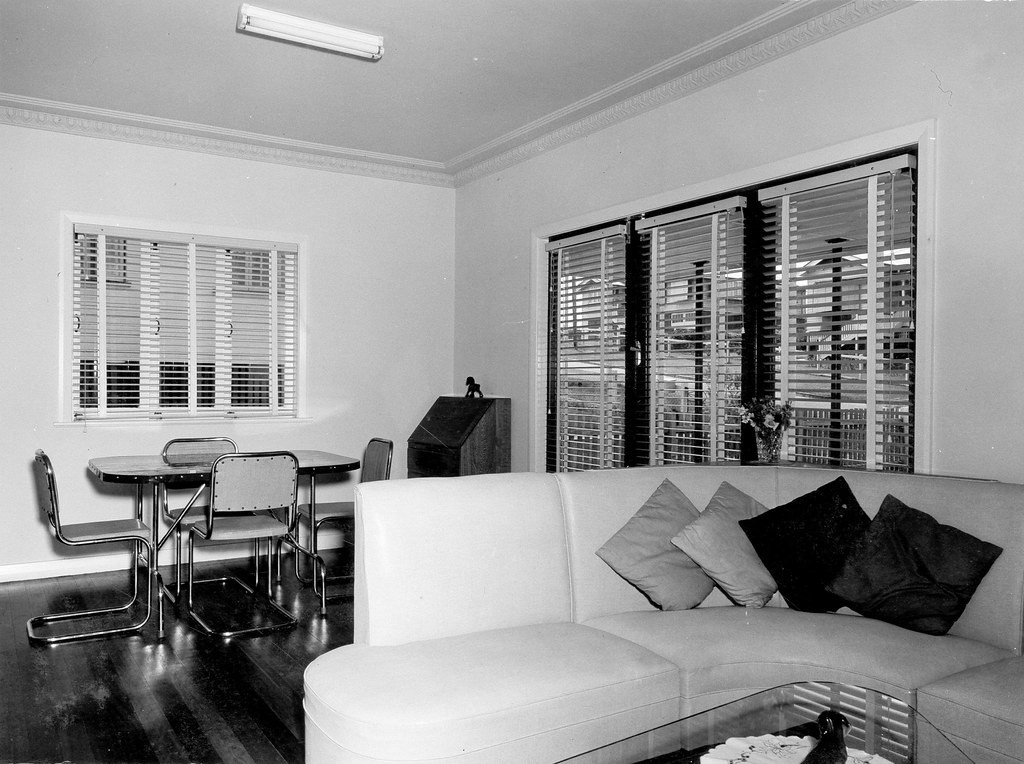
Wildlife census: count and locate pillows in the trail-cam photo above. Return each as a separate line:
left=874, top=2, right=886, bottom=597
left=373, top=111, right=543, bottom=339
left=670, top=482, right=778, bottom=609
left=824, top=494, right=1004, bottom=636
left=738, top=476, right=871, bottom=615
left=594, top=478, right=717, bottom=611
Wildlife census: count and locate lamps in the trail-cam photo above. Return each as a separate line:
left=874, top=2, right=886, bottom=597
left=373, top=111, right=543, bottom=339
left=237, top=3, right=385, bottom=59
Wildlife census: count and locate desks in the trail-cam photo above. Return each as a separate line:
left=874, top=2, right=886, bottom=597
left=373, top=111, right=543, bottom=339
left=89, top=450, right=361, bottom=643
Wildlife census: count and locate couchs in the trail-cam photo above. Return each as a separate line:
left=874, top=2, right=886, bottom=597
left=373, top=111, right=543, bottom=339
left=299, top=462, right=1024, bottom=764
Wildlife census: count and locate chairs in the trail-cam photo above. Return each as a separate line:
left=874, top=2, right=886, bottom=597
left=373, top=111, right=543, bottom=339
left=188, top=451, right=299, bottom=637
left=296, top=438, right=394, bottom=600
left=27, top=449, right=153, bottom=644
left=162, top=437, right=260, bottom=605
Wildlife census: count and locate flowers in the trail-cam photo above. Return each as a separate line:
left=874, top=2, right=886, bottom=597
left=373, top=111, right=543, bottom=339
left=736, top=396, right=794, bottom=432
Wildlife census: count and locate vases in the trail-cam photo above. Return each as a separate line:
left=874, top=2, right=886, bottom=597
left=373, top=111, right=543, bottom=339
left=755, top=427, right=783, bottom=462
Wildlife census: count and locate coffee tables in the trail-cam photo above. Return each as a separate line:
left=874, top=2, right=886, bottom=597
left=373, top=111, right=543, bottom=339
left=554, top=680, right=975, bottom=764
